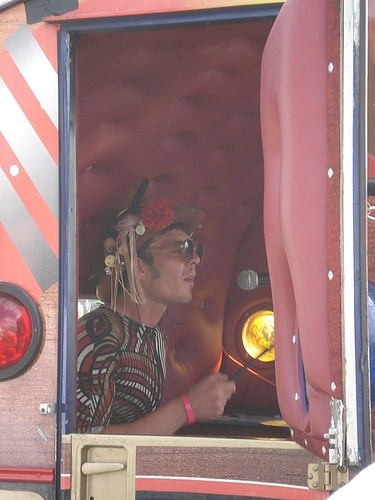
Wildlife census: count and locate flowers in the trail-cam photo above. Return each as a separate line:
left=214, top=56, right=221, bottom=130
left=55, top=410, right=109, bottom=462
left=142, top=197, right=175, bottom=232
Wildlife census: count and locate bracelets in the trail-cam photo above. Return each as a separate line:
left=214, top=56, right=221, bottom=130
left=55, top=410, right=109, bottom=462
left=180, top=395, right=194, bottom=424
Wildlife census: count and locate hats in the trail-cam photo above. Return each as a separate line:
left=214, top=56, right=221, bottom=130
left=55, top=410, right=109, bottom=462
left=93, top=203, right=207, bottom=306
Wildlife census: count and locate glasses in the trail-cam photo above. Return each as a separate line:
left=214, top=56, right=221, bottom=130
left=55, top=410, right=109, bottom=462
left=139, top=236, right=203, bottom=261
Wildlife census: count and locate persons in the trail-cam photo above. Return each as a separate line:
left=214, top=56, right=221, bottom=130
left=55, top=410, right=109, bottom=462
left=78, top=178, right=237, bottom=436
